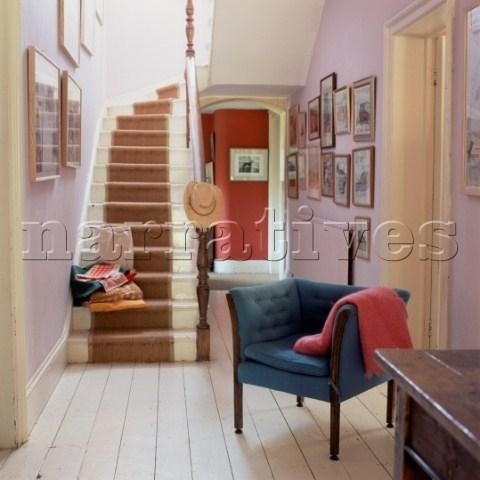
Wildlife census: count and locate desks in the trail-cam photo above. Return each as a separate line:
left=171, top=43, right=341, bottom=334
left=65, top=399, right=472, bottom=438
left=374, top=345, right=480, bottom=480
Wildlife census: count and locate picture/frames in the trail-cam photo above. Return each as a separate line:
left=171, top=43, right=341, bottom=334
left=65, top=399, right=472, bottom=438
left=295, top=148, right=309, bottom=196
left=305, top=144, right=322, bottom=202
left=96, top=0, right=105, bottom=26
left=307, top=96, right=321, bottom=141
left=295, top=111, right=306, bottom=150
left=333, top=153, right=351, bottom=209
left=351, top=146, right=375, bottom=209
left=287, top=103, right=301, bottom=146
left=58, top=0, right=81, bottom=67
left=286, top=151, right=299, bottom=200
left=461, top=50, right=480, bottom=197
left=331, top=84, right=352, bottom=136
left=319, top=151, right=334, bottom=198
left=350, top=74, right=375, bottom=144
left=317, top=72, right=337, bottom=149
left=79, top=1, right=96, bottom=55
left=353, top=215, right=371, bottom=261
left=226, top=145, right=270, bottom=183
left=62, top=70, right=83, bottom=169
left=26, top=45, right=64, bottom=181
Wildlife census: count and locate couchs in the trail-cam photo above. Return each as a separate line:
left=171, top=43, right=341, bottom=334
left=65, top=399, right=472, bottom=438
left=224, top=275, right=410, bottom=461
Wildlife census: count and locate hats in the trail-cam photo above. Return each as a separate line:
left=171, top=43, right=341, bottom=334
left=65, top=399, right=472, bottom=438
left=184, top=181, right=224, bottom=229
